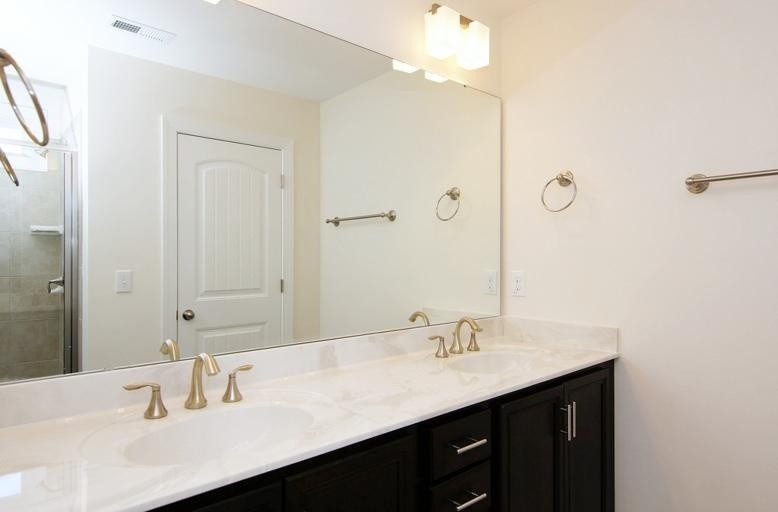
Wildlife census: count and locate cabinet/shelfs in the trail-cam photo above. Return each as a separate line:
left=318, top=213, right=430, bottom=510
left=279, top=414, right=415, bottom=511
left=147, top=470, right=279, bottom=511
left=498, top=362, right=616, bottom=512
left=416, top=407, right=498, bottom=512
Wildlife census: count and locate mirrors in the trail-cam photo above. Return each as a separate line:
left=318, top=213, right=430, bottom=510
left=1, top=0, right=502, bottom=384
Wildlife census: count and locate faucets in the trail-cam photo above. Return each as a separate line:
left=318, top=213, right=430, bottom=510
left=184, top=351, right=221, bottom=410
left=448, top=317, right=481, bottom=354
left=408, top=310, right=430, bottom=326
left=159, top=337, right=181, bottom=362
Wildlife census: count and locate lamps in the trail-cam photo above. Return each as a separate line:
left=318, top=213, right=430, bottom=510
left=424, top=2, right=490, bottom=69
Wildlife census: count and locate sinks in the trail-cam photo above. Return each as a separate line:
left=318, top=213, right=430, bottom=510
left=445, top=349, right=536, bottom=377
left=121, top=401, right=319, bottom=469
